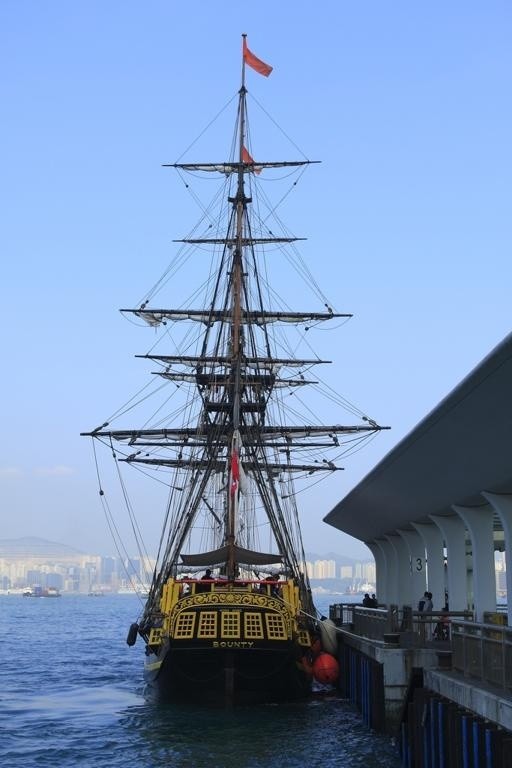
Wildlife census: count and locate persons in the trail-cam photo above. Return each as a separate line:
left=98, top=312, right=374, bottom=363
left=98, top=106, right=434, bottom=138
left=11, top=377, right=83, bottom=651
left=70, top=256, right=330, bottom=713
left=418, top=591, right=428, bottom=613
left=200, top=569, right=218, bottom=591
left=373, top=594, right=378, bottom=608
left=362, top=593, right=374, bottom=606
left=260, top=575, right=281, bottom=592
left=445, top=593, right=448, bottom=603
left=423, top=592, right=434, bottom=641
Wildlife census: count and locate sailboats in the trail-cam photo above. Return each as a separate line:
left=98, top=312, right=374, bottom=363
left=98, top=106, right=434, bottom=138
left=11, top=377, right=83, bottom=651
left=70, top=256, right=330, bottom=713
left=79, top=33, right=391, bottom=712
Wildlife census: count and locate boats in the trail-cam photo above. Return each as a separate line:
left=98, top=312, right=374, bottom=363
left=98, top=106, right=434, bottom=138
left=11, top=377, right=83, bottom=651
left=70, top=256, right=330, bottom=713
left=23, top=587, right=60, bottom=597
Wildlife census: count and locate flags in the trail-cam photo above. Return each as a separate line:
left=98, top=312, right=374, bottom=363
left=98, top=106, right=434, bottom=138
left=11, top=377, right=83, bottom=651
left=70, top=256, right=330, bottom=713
left=229, top=447, right=239, bottom=498
left=244, top=38, right=273, bottom=77
left=241, top=148, right=264, bottom=179
